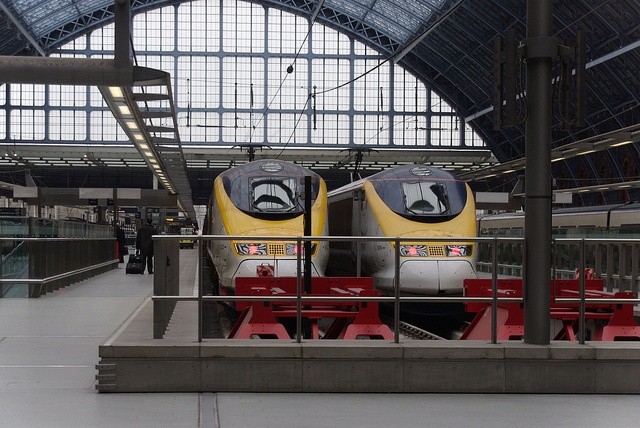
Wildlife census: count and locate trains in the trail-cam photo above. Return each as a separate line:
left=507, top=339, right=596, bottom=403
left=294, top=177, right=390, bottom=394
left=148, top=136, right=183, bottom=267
left=326, top=165, right=476, bottom=298
left=476, top=201, right=640, bottom=292
left=202, top=159, right=330, bottom=313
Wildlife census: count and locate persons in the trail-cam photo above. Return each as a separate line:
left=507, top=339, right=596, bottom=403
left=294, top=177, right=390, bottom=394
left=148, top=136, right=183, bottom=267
left=135, top=219, right=156, bottom=275
left=114, top=224, right=127, bottom=264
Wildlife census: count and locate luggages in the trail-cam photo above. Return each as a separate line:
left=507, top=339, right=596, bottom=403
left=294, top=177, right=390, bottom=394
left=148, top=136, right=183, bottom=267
left=126, top=248, right=146, bottom=275
